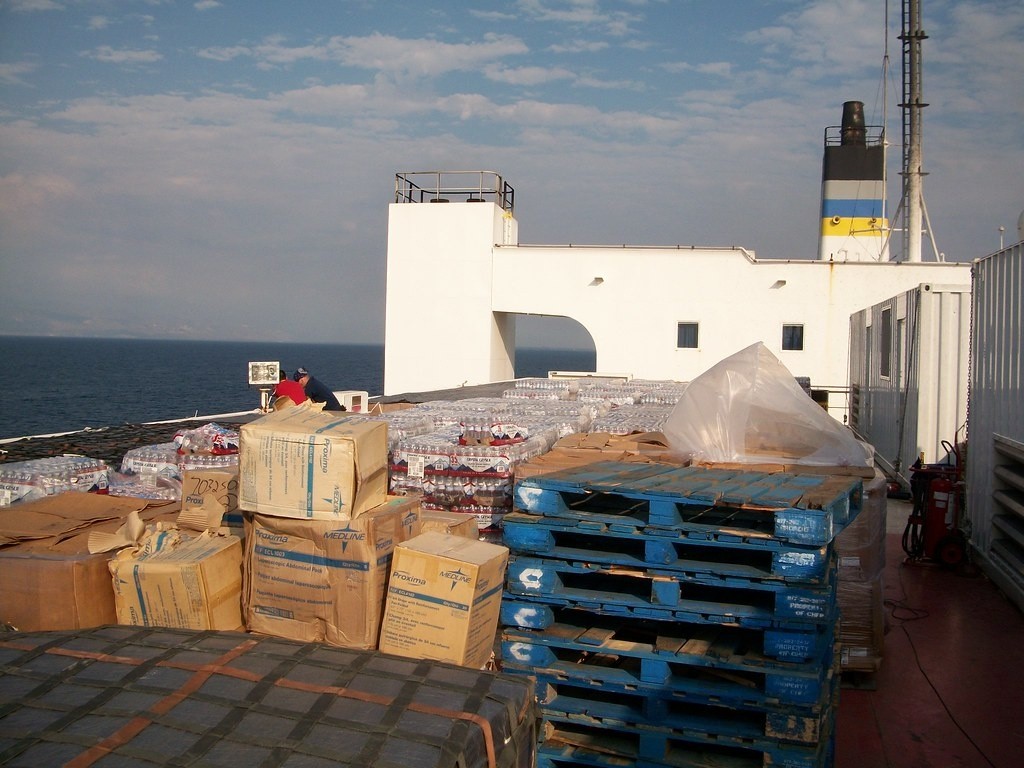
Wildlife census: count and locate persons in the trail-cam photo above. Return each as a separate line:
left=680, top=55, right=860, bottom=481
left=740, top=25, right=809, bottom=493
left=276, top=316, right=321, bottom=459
left=273, top=367, right=346, bottom=411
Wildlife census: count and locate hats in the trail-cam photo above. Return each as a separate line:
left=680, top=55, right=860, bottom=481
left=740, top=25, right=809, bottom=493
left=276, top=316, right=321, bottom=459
left=293, top=368, right=308, bottom=383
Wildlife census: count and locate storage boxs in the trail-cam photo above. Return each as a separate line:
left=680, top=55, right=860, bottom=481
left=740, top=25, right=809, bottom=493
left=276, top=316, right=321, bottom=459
left=0, top=490, right=182, bottom=635
left=244, top=495, right=422, bottom=651
left=427, top=511, right=480, bottom=544
left=176, top=467, right=246, bottom=527
left=88, top=510, right=251, bottom=628
left=380, top=531, right=508, bottom=670
left=238, top=406, right=388, bottom=520
